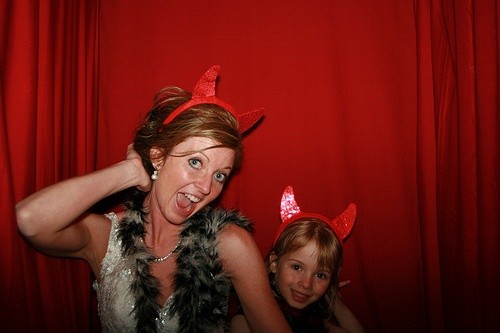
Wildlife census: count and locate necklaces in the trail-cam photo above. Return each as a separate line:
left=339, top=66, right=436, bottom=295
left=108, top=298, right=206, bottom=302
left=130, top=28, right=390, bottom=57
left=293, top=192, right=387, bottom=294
left=151, top=240, right=180, bottom=263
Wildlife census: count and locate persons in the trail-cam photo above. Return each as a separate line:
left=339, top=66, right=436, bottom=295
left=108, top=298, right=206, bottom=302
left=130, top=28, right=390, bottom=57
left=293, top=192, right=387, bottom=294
left=15, top=65, right=293, bottom=333
left=230, top=215, right=364, bottom=333
